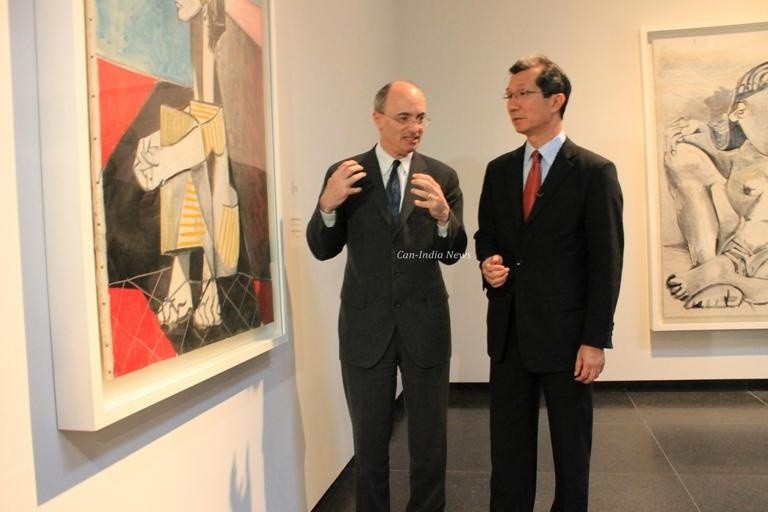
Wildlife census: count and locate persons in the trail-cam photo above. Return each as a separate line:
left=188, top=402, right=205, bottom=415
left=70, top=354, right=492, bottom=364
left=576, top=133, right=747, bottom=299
left=665, top=59, right=766, bottom=308
left=134, top=1, right=241, bottom=329
left=306, top=79, right=468, bottom=512
left=474, top=56, right=624, bottom=512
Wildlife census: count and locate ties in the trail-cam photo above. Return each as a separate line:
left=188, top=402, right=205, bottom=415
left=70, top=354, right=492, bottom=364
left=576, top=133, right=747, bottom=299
left=523, top=150, right=541, bottom=222
left=387, top=160, right=401, bottom=216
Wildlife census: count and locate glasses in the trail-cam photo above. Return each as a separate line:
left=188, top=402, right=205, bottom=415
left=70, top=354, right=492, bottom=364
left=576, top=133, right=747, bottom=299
left=377, top=109, right=432, bottom=128
left=503, top=89, right=541, bottom=102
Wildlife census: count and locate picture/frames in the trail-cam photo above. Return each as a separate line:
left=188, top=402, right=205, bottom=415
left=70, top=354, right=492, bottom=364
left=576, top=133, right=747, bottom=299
left=637, top=20, right=768, bottom=333
left=27, top=1, right=293, bottom=434
